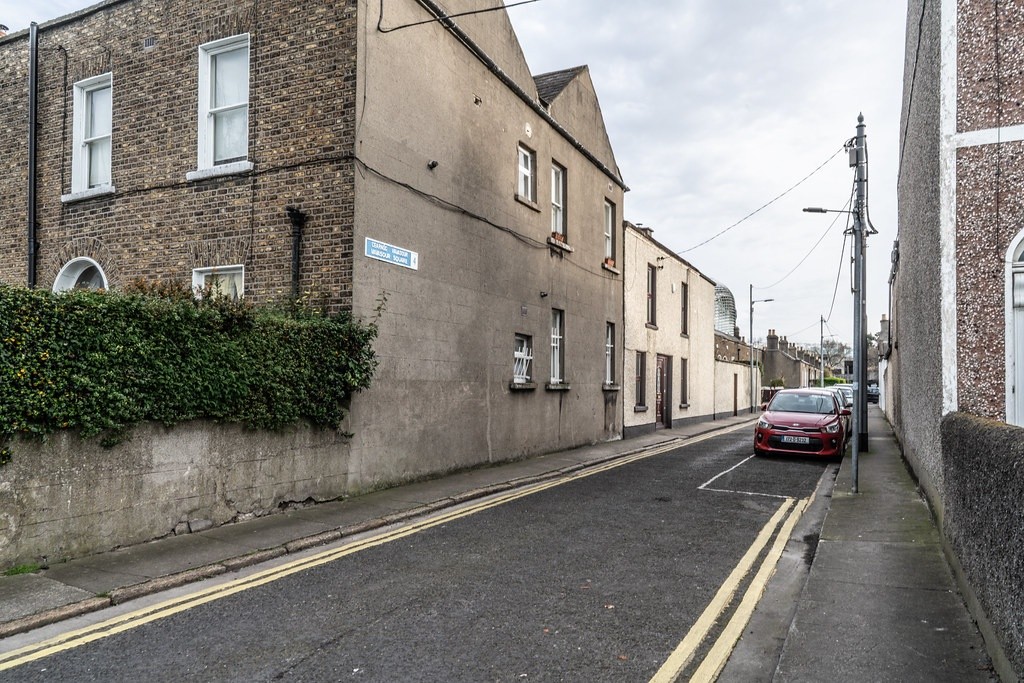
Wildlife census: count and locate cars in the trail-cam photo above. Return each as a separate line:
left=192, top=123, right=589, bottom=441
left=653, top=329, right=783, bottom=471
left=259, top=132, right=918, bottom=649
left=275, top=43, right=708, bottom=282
left=792, top=383, right=853, bottom=432
left=753, top=388, right=852, bottom=463
left=867, top=386, right=879, bottom=404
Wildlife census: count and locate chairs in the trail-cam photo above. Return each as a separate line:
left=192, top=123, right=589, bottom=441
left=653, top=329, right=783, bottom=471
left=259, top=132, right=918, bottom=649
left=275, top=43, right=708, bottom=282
left=782, top=396, right=800, bottom=410
left=815, top=398, right=831, bottom=411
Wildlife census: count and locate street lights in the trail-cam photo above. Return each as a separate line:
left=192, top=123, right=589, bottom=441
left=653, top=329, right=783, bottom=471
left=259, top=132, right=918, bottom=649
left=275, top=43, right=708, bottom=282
left=748, top=284, right=776, bottom=413
left=802, top=206, right=869, bottom=452
left=820, top=314, right=835, bottom=388
